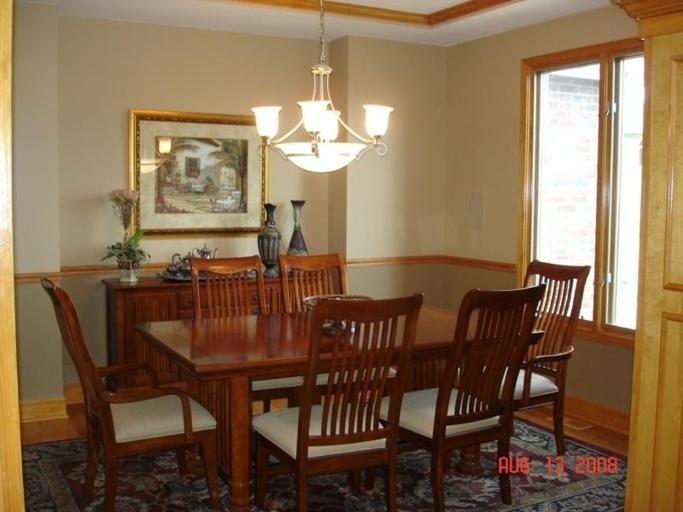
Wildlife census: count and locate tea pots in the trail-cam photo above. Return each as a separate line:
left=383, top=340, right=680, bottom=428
left=172, top=244, right=218, bottom=277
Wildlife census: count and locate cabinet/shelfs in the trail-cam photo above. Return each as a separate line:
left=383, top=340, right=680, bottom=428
left=100, top=269, right=330, bottom=391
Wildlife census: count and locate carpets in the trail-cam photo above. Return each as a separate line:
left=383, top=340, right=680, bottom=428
left=20, top=413, right=627, bottom=510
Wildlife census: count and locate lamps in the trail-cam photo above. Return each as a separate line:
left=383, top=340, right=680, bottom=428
left=252, top=0, right=395, bottom=175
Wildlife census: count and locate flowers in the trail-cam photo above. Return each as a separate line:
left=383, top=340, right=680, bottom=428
left=93, top=187, right=152, bottom=262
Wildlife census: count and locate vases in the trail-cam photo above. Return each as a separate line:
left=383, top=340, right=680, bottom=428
left=117, top=256, right=140, bottom=284
left=256, top=197, right=310, bottom=277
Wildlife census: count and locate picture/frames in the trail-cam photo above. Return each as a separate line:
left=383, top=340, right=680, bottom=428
left=125, top=105, right=267, bottom=237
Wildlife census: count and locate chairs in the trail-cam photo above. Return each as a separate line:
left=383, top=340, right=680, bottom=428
left=278, top=253, right=358, bottom=400
left=246, top=290, right=428, bottom=512
left=187, top=255, right=270, bottom=423
left=368, top=280, right=548, bottom=512
left=430, top=257, right=591, bottom=458
left=40, top=275, right=226, bottom=510
